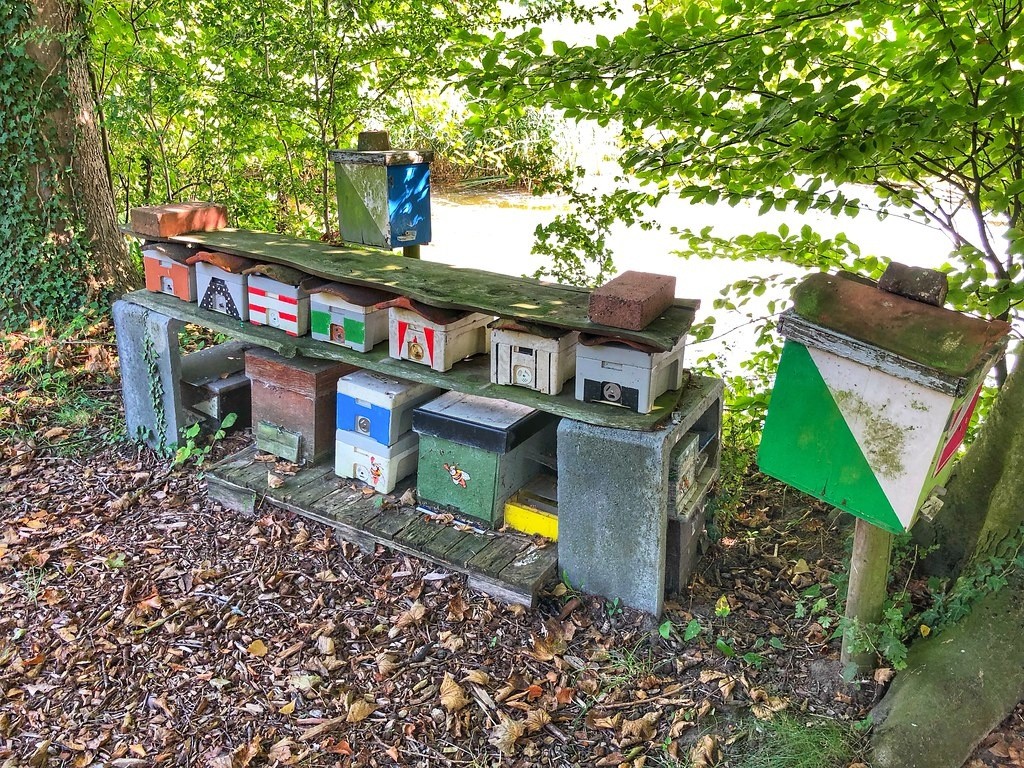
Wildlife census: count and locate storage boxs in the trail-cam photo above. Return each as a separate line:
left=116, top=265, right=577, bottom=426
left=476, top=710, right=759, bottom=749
left=143, top=250, right=198, bottom=303
left=410, top=390, right=558, bottom=529
left=575, top=334, right=686, bottom=412
left=388, top=306, right=495, bottom=373
left=243, top=347, right=360, bottom=463
left=195, top=261, right=249, bottom=323
left=249, top=273, right=312, bottom=336
left=335, top=428, right=420, bottom=494
left=336, top=368, right=441, bottom=445
left=180, top=372, right=252, bottom=436
left=489, top=325, right=580, bottom=395
left=310, top=292, right=389, bottom=352
